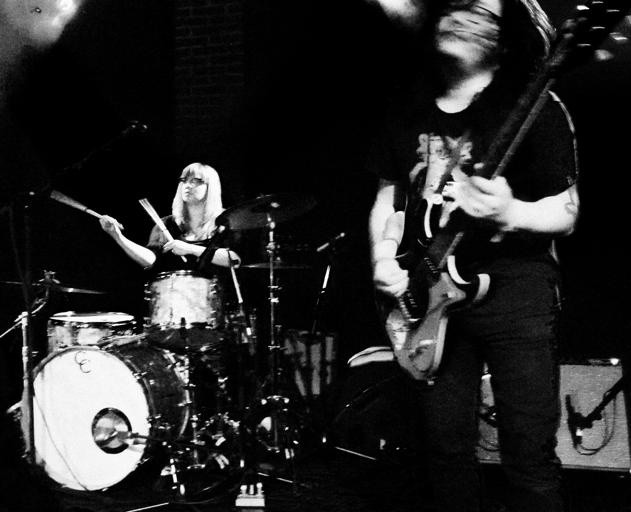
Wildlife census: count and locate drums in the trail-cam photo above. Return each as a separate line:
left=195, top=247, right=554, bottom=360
left=143, top=270, right=226, bottom=327
left=47, top=311, right=136, bottom=354
left=15, top=346, right=196, bottom=494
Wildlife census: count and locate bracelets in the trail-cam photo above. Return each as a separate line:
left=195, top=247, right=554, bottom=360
left=368, top=236, right=400, bottom=265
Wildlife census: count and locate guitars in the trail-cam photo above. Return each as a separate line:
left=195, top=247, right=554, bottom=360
left=381, top=1, right=631, bottom=388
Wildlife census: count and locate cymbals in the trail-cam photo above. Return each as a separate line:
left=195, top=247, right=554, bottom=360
left=215, top=192, right=320, bottom=231
left=2, top=279, right=104, bottom=295
left=240, top=262, right=314, bottom=271
left=149, top=322, right=233, bottom=353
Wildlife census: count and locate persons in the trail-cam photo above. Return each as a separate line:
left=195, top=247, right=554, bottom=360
left=98, top=160, right=244, bottom=330
left=369, top=1, right=582, bottom=511
